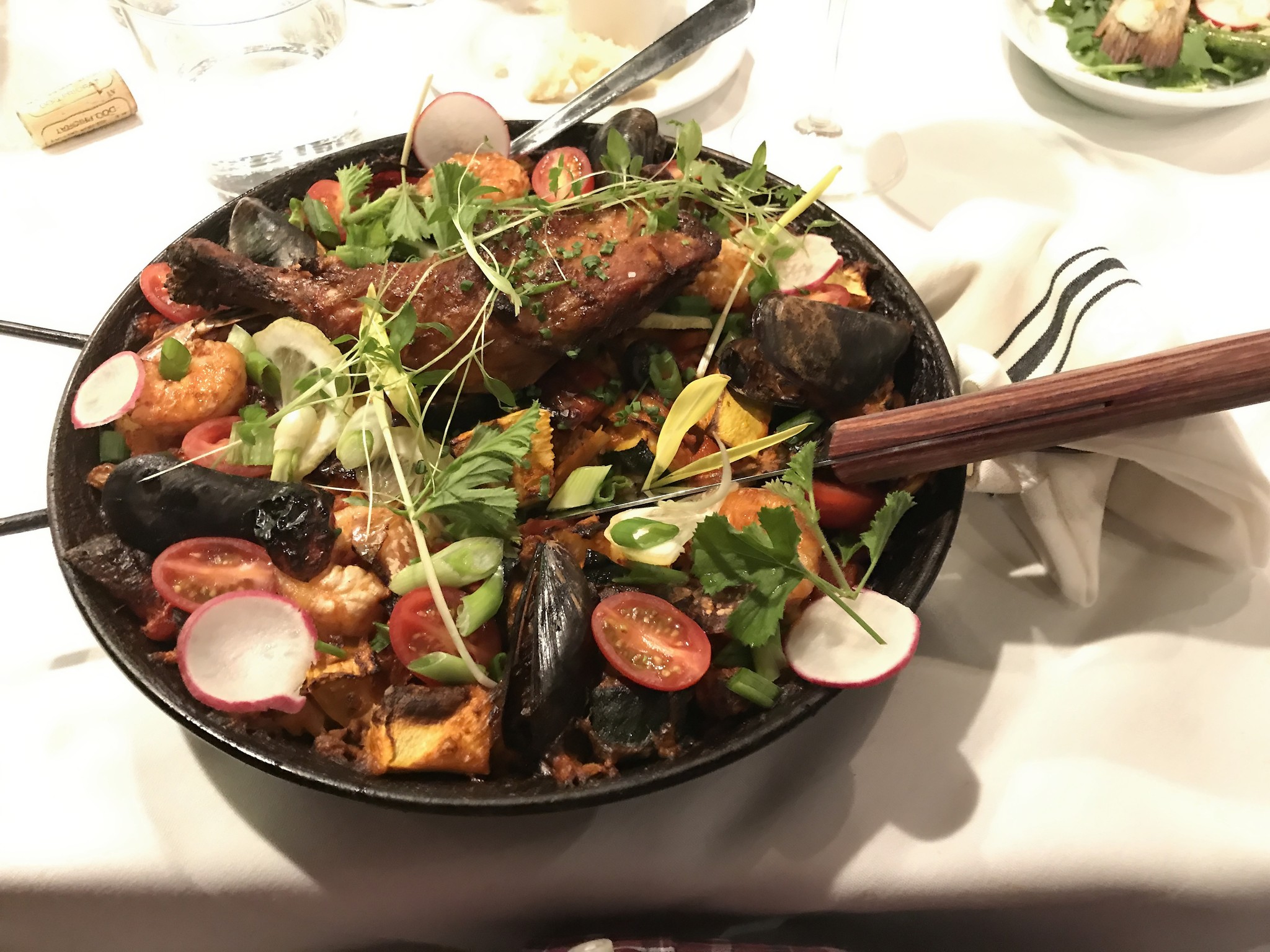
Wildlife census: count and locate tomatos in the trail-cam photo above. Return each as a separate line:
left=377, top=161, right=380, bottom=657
left=68, top=94, right=917, bottom=786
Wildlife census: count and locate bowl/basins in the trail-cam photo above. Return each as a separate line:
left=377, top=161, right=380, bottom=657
left=43, top=117, right=971, bottom=814
left=1001, top=0, right=1269, bottom=121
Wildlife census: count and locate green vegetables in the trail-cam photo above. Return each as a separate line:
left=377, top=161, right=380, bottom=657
left=1041, top=0, right=1270, bottom=89
